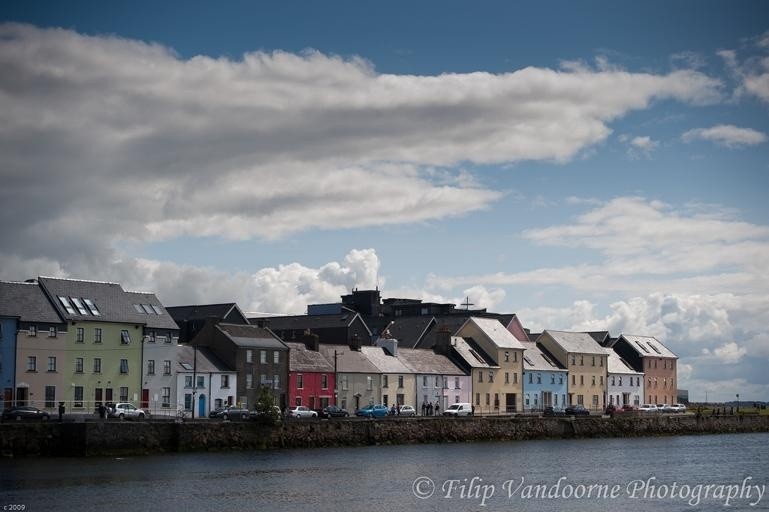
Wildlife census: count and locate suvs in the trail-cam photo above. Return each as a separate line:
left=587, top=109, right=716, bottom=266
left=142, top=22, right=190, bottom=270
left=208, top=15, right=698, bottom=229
left=106, top=403, right=147, bottom=420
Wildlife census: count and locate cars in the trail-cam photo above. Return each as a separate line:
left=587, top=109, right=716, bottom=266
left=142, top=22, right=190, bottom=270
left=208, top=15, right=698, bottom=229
left=543, top=405, right=591, bottom=415
left=284, top=405, right=319, bottom=419
left=356, top=404, right=390, bottom=418
left=2, top=405, right=51, bottom=422
left=390, top=404, right=416, bottom=416
left=249, top=406, right=282, bottom=420
left=318, top=405, right=350, bottom=418
left=607, top=404, right=686, bottom=414
left=209, top=405, right=250, bottom=421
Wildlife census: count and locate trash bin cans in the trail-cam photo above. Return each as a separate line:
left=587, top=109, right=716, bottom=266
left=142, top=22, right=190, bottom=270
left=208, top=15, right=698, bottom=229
left=544, top=407, right=554, bottom=416
left=624, top=407, right=634, bottom=416
left=58, top=403, right=66, bottom=418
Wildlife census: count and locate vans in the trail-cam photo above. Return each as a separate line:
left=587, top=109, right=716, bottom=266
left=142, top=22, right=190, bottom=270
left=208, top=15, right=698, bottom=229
left=442, top=403, right=473, bottom=417
